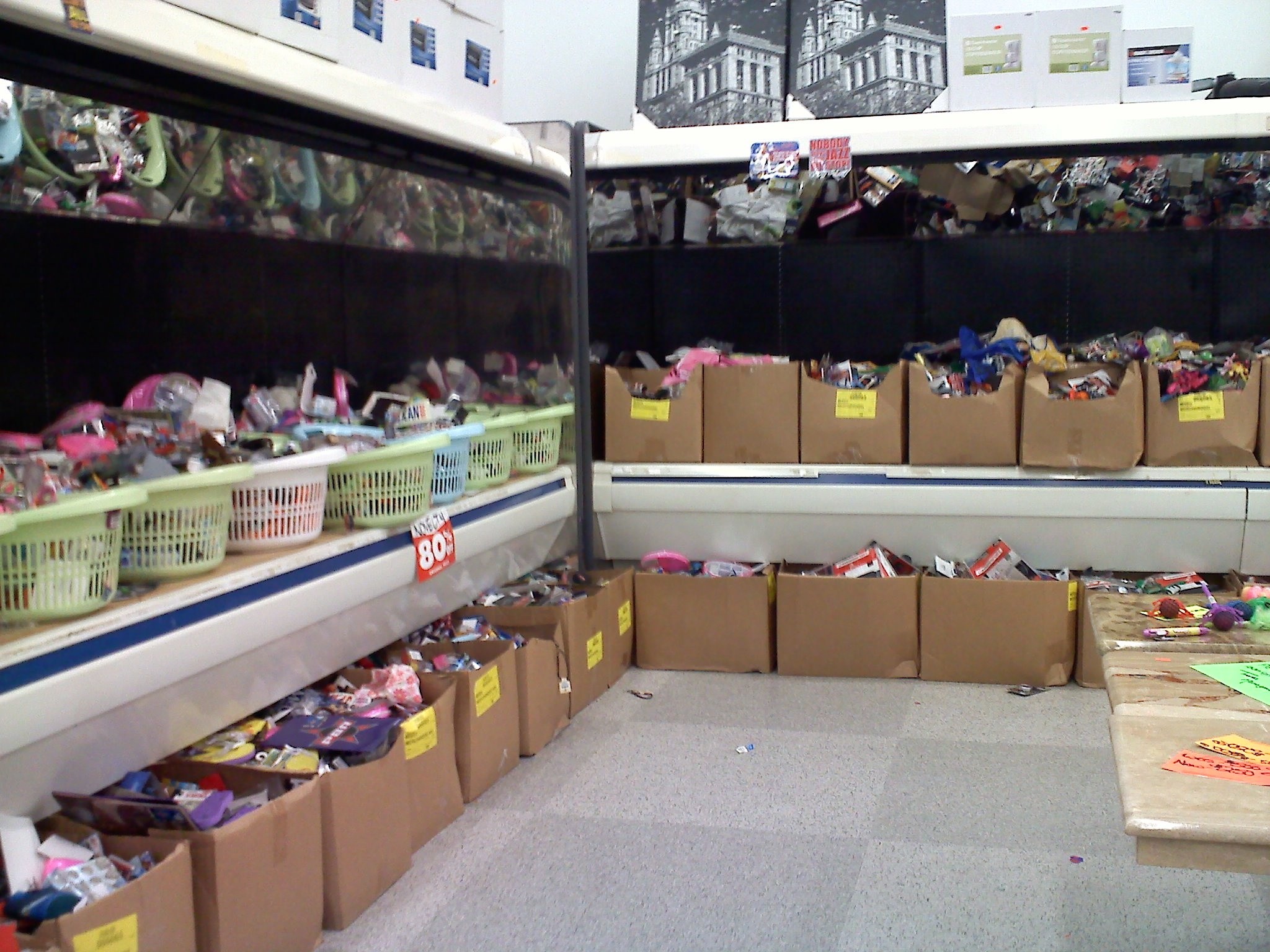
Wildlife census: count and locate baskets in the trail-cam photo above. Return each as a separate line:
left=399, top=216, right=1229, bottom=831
left=2, top=483, right=150, bottom=619
left=325, top=433, right=451, bottom=525
left=498, top=400, right=575, bottom=469
left=120, top=463, right=255, bottom=576
left=452, top=409, right=528, bottom=485
left=228, top=445, right=346, bottom=549
left=390, top=423, right=484, bottom=504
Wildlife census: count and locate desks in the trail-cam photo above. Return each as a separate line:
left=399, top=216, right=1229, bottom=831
left=1086, top=592, right=1268, bottom=655
left=1109, top=713, right=1270, bottom=876
left=1103, top=648, right=1270, bottom=718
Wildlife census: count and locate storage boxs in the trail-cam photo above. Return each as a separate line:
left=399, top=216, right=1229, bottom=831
left=1, top=357, right=1270, bottom=952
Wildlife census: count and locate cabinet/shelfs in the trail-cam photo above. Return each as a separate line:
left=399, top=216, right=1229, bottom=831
left=0, top=0, right=588, bottom=840
left=576, top=91, right=1270, bottom=580
